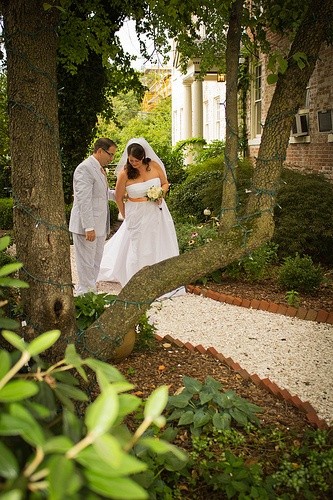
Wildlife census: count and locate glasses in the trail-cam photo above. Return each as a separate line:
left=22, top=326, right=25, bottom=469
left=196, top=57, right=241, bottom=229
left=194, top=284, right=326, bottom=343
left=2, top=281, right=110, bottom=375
left=102, top=148, right=114, bottom=159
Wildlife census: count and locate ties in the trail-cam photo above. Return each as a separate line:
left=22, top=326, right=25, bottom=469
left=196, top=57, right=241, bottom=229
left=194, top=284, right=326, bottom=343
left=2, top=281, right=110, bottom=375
left=101, top=168, right=109, bottom=188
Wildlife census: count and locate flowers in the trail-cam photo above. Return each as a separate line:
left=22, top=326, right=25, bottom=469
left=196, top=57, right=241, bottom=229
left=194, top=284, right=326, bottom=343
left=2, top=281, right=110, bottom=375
left=144, top=185, right=165, bottom=211
left=104, top=168, right=109, bottom=174
